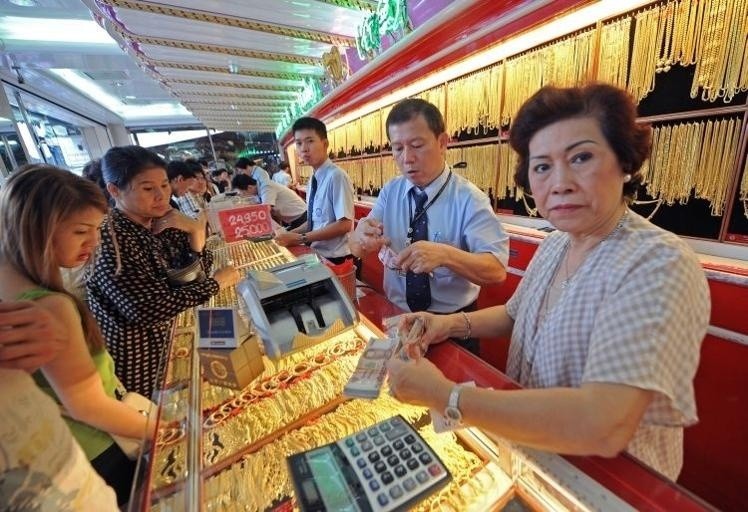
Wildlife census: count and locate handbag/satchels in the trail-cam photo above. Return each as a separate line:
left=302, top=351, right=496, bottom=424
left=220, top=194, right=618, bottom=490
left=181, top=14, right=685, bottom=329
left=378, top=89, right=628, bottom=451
left=166, top=257, right=202, bottom=287
left=108, top=390, right=158, bottom=460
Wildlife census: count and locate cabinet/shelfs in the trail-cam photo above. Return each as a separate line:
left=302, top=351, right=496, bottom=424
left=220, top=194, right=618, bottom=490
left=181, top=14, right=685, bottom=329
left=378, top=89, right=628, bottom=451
left=127, top=193, right=747, bottom=511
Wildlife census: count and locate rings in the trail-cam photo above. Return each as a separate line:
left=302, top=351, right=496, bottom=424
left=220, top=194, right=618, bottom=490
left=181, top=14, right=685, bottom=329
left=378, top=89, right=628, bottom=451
left=162, top=218, right=168, bottom=224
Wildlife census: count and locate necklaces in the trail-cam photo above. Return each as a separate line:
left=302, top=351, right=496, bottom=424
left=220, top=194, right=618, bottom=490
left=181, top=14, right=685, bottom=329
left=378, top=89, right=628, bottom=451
left=300, top=0, right=748, bottom=222
left=560, top=208, right=633, bottom=294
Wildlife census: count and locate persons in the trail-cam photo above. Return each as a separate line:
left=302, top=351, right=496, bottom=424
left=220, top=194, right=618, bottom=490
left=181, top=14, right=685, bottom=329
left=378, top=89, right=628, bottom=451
left=1, top=164, right=161, bottom=510
left=386, top=82, right=711, bottom=484
left=273, top=117, right=361, bottom=278
left=345, top=98, right=512, bottom=359
left=87, top=145, right=241, bottom=400
left=81, top=152, right=309, bottom=227
left=1, top=300, right=121, bottom=512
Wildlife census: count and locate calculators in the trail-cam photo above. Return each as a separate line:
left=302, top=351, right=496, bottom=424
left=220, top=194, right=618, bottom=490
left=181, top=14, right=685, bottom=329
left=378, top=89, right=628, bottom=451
left=287, top=415, right=452, bottom=512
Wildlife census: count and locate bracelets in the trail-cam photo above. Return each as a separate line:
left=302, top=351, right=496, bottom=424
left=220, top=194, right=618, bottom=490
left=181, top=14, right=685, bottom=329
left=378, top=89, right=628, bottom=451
left=456, top=309, right=472, bottom=341
left=430, top=464, right=495, bottom=511
left=150, top=237, right=495, bottom=512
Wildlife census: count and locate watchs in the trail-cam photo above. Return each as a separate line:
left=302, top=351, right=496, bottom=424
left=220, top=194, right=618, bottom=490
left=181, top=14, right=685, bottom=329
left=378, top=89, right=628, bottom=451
left=443, top=382, right=465, bottom=426
left=301, top=232, right=310, bottom=245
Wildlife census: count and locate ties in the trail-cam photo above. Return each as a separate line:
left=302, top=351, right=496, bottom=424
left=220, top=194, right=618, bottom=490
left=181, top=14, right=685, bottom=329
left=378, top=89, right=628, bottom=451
left=405, top=186, right=432, bottom=312
left=306, top=175, right=317, bottom=245
